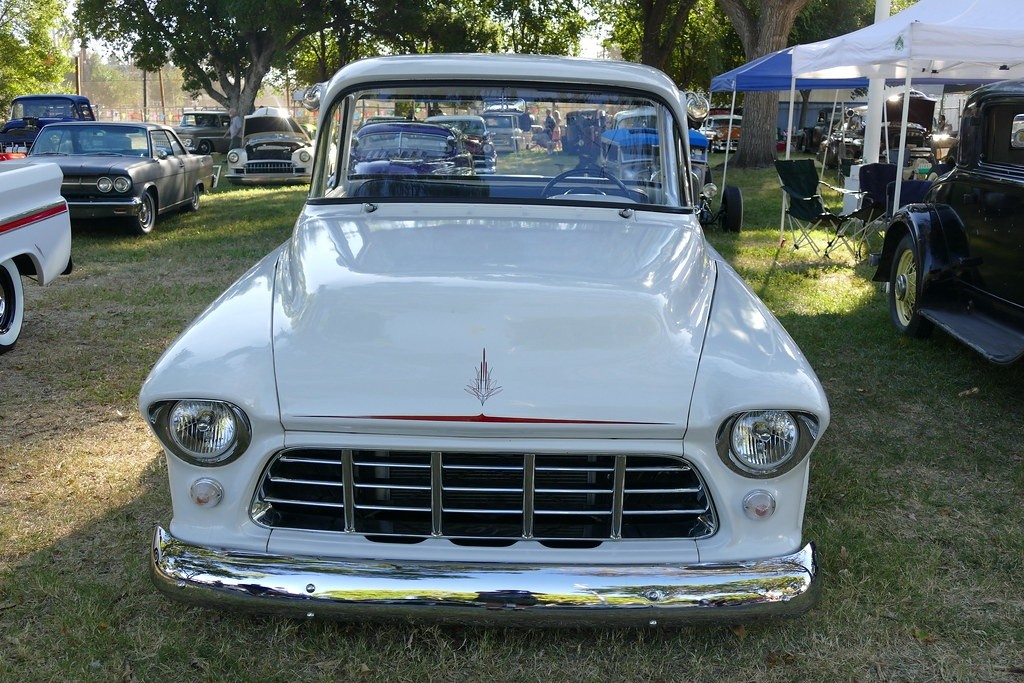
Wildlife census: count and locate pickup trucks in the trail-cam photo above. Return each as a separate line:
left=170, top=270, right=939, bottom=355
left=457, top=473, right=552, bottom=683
left=0, top=158, right=74, bottom=356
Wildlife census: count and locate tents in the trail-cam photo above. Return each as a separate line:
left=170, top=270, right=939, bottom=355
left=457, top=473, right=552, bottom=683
left=706, top=0, right=1024, bottom=247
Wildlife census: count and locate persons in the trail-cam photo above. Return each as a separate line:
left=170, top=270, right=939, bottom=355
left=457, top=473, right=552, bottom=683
left=545, top=109, right=563, bottom=149
left=406, top=110, right=417, bottom=120
left=938, top=115, right=945, bottom=131
left=519, top=107, right=531, bottom=149
left=535, top=126, right=553, bottom=155
left=430, top=109, right=447, bottom=116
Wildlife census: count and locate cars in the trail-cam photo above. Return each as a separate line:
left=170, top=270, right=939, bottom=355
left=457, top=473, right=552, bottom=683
left=872, top=77, right=1023, bottom=369
left=152, top=106, right=230, bottom=156
left=793, top=87, right=938, bottom=177
left=560, top=108, right=612, bottom=155
left=224, top=97, right=529, bottom=191
left=137, top=47, right=832, bottom=629
left=26, top=121, right=216, bottom=235
left=597, top=108, right=743, bottom=234
left=0, top=94, right=96, bottom=158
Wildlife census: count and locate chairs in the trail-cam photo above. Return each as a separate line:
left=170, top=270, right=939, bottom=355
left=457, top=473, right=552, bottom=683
left=773, top=155, right=934, bottom=260
